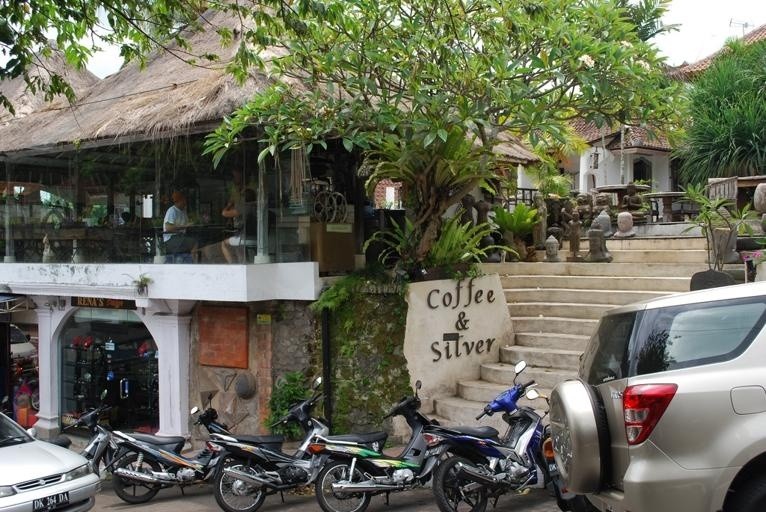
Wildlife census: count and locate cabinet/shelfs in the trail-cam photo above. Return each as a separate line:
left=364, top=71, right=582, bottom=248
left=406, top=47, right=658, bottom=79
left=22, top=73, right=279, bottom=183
left=60, top=340, right=156, bottom=435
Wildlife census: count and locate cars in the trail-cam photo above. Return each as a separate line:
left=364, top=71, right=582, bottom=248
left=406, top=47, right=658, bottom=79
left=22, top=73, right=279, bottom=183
left=0, top=407, right=102, bottom=512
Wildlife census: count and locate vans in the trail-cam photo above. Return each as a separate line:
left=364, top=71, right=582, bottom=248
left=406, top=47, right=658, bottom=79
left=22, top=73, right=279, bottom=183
left=8, top=322, right=39, bottom=374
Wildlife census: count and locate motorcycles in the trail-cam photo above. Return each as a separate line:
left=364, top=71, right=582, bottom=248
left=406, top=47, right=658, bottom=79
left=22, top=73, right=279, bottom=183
left=304, top=378, right=476, bottom=512
left=41, top=401, right=133, bottom=488
left=408, top=359, right=559, bottom=512
left=203, top=374, right=365, bottom=512
left=96, top=390, right=241, bottom=506
left=11, top=370, right=39, bottom=412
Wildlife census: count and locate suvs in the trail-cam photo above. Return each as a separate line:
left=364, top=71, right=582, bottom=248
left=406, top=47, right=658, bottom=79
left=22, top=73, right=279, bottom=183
left=545, top=278, right=766, bottom=512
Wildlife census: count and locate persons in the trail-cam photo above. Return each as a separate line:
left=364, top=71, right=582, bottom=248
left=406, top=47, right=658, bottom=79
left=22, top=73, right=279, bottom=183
left=163, top=189, right=201, bottom=264
left=219, top=190, right=260, bottom=262
left=542, top=234, right=560, bottom=257
left=615, top=182, right=645, bottom=219
left=221, top=166, right=258, bottom=238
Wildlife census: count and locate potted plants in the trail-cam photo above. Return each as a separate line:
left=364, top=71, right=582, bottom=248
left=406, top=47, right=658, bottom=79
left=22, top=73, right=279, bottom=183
left=361, top=207, right=520, bottom=281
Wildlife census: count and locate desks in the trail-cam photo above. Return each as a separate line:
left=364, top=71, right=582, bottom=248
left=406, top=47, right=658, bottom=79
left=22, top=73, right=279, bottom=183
left=176, top=223, right=234, bottom=263
left=643, top=191, right=687, bottom=225
